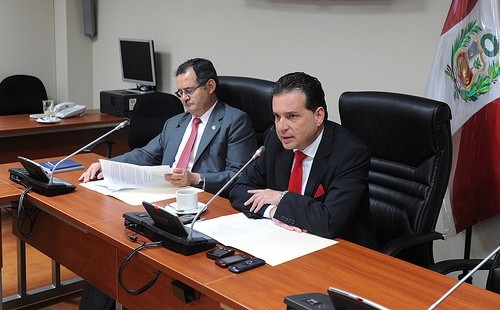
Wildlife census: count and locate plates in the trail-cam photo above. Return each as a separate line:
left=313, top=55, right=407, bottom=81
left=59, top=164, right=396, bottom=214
left=165, top=202, right=207, bottom=214
left=36, top=118, right=61, bottom=123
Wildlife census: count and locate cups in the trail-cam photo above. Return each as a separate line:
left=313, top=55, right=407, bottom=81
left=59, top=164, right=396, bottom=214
left=42, top=100, right=54, bottom=119
left=176, top=189, right=198, bottom=210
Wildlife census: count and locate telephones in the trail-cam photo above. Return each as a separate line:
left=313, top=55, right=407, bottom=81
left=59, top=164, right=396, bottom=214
left=52, top=101, right=86, bottom=118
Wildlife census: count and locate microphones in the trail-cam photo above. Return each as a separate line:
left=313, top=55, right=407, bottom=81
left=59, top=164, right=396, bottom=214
left=8, top=119, right=130, bottom=197
left=121, top=143, right=266, bottom=255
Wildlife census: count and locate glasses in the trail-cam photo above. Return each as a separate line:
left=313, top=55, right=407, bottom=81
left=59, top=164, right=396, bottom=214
left=175, top=83, right=206, bottom=98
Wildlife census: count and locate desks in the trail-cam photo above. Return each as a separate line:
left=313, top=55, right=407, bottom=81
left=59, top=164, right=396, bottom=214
left=0, top=108, right=500, bottom=310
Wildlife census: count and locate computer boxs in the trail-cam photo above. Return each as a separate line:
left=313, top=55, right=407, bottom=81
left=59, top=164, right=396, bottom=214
left=99, top=88, right=161, bottom=116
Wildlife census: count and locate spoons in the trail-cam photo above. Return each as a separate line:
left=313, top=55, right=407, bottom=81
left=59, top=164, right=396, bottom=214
left=166, top=203, right=184, bottom=213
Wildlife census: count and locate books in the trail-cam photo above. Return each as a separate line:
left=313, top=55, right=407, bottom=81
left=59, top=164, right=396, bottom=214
left=39, top=159, right=85, bottom=175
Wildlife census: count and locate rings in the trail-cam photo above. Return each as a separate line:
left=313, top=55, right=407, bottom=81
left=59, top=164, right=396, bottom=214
left=177, top=180, right=179, bottom=184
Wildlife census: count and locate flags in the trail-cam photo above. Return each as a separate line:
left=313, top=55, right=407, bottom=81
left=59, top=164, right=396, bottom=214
left=423, top=0, right=500, bottom=238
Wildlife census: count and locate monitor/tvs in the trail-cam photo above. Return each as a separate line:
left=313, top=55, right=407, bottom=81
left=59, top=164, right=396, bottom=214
left=117, top=37, right=156, bottom=94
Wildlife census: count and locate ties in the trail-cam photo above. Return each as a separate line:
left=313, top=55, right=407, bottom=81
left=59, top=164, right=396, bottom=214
left=176, top=118, right=202, bottom=169
left=288, top=150, right=308, bottom=195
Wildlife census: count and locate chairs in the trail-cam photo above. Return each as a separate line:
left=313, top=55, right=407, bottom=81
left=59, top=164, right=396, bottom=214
left=338, top=91, right=453, bottom=267
left=215, top=74, right=277, bottom=150
left=128, top=90, right=185, bottom=151
left=0, top=74, right=49, bottom=115
left=429, top=246, right=500, bottom=294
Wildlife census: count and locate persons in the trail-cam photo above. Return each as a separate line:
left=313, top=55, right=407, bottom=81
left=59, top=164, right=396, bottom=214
left=230, top=72, right=371, bottom=239
left=78, top=57, right=256, bottom=310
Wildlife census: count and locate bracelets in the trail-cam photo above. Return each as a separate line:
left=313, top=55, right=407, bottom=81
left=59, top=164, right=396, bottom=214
left=279, top=191, right=288, bottom=200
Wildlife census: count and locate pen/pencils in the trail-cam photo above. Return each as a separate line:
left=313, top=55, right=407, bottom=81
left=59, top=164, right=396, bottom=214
left=48, top=162, right=55, bottom=167
left=45, top=163, right=53, bottom=169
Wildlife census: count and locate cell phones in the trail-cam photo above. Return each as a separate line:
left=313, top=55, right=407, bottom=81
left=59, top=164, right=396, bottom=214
left=178, top=214, right=200, bottom=225
left=228, top=257, right=266, bottom=273
left=206, top=246, right=235, bottom=260
left=214, top=253, right=250, bottom=269
